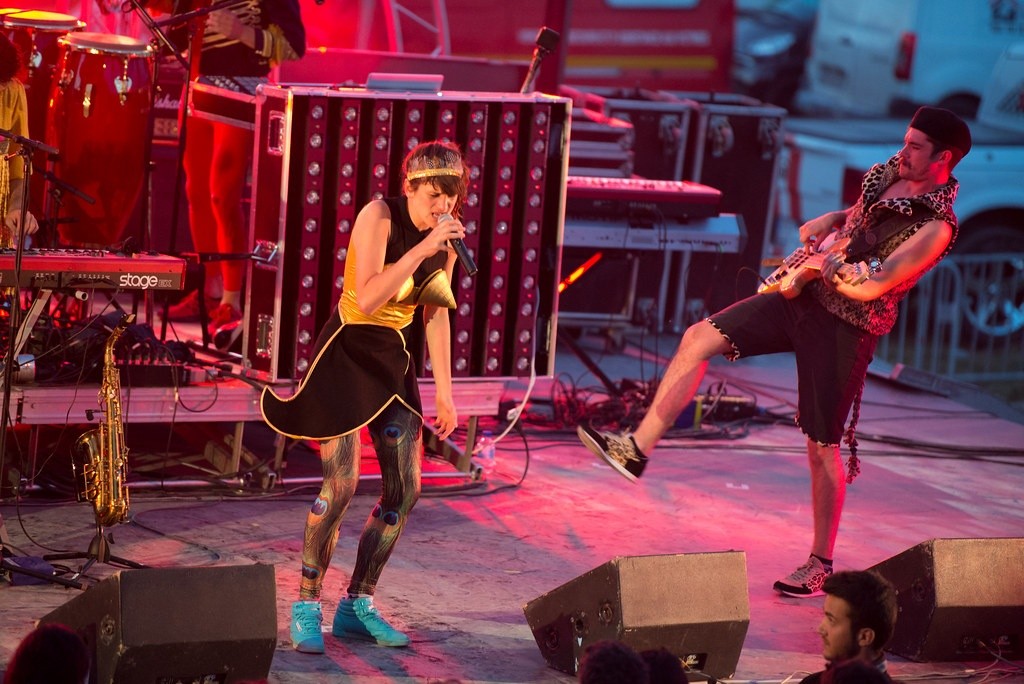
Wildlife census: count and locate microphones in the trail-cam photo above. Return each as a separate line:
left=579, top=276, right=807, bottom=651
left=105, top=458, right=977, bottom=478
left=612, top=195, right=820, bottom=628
left=439, top=216, right=478, bottom=276
left=84, top=409, right=96, bottom=421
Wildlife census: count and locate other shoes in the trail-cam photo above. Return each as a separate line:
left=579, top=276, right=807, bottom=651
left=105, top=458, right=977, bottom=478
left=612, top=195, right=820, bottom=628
left=155, top=286, right=242, bottom=341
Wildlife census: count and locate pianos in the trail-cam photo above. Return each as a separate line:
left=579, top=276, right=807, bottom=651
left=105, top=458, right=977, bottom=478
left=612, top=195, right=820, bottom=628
left=0, top=248, right=188, bottom=291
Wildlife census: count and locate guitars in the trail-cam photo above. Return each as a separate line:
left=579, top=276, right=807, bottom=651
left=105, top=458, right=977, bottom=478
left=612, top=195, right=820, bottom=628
left=755, top=229, right=883, bottom=300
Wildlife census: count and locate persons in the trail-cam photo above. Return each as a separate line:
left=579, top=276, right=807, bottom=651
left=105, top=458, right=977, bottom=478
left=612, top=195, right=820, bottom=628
left=5, top=622, right=92, bottom=684
left=151, top=0, right=305, bottom=343
left=0, top=32, right=39, bottom=247
left=577, top=104, right=972, bottom=597
left=578, top=572, right=904, bottom=684
left=260, top=143, right=472, bottom=651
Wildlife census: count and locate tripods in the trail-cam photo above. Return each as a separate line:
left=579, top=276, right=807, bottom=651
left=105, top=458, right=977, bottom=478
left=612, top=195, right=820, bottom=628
left=43, top=523, right=155, bottom=591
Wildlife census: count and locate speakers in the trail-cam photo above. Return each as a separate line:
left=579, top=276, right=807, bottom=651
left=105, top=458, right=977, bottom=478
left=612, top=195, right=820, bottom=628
left=868, top=537, right=1024, bottom=662
left=36, top=564, right=278, bottom=684
left=523, top=549, right=749, bottom=682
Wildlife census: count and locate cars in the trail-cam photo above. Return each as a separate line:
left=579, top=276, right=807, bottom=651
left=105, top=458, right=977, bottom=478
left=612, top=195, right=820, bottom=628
left=736, top=1, right=821, bottom=98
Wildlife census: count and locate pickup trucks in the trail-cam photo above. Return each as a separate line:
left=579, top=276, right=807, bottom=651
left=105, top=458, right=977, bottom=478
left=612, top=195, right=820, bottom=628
left=776, top=39, right=1024, bottom=351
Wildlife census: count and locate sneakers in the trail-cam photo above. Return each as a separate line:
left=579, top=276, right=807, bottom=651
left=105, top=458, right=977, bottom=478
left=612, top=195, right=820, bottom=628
left=332, top=598, right=410, bottom=646
left=577, top=425, right=650, bottom=483
left=290, top=601, right=325, bottom=652
left=771, top=555, right=833, bottom=598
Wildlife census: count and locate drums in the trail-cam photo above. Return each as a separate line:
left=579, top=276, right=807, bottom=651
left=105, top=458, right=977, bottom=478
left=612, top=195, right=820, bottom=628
left=0, top=9, right=154, bottom=250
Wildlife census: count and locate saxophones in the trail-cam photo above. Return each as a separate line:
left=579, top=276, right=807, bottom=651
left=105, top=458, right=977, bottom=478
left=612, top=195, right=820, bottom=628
left=75, top=311, right=137, bottom=528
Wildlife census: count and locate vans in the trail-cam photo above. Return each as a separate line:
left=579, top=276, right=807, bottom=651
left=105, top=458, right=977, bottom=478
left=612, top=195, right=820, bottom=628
left=797, top=0, right=1023, bottom=116
